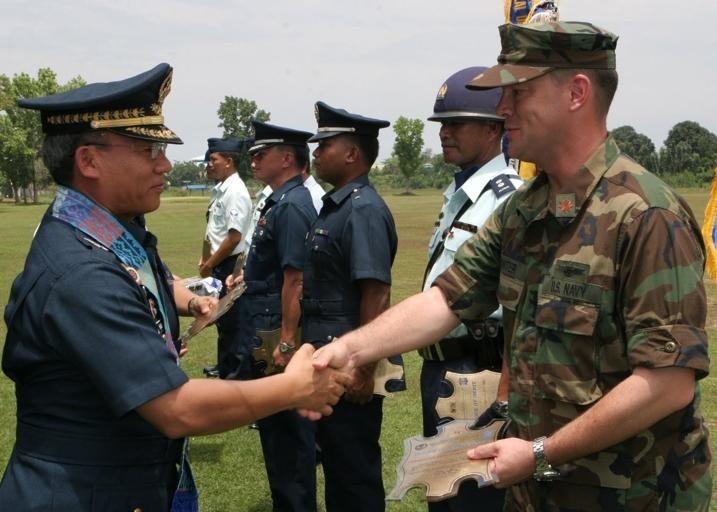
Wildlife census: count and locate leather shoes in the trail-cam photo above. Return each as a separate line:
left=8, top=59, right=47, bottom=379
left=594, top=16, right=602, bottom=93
left=203, top=367, right=215, bottom=372
left=207, top=370, right=220, bottom=376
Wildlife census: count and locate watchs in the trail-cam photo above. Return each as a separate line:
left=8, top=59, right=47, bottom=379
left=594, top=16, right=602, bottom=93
left=532, top=435, right=569, bottom=496
left=278, top=340, right=297, bottom=355
left=492, top=400, right=512, bottom=420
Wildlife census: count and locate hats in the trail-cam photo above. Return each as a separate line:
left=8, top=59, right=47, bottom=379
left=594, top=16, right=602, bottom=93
left=208, top=138, right=244, bottom=152
left=307, top=101, right=391, bottom=144
left=247, top=120, right=314, bottom=152
left=464, top=21, right=619, bottom=91
left=17, top=63, right=183, bottom=145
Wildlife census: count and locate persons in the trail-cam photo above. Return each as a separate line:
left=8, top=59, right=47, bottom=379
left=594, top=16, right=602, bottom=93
left=297, top=22, right=714, bottom=512
left=418, top=66, right=526, bottom=510
left=226, top=140, right=274, bottom=298
left=1, top=63, right=355, bottom=512
left=299, top=67, right=399, bottom=512
left=296, top=141, right=326, bottom=218
left=240, top=119, right=319, bottom=512
left=198, top=138, right=254, bottom=378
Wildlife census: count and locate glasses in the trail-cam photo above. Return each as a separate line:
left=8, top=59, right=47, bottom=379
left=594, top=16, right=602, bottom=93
left=83, top=142, right=168, bottom=159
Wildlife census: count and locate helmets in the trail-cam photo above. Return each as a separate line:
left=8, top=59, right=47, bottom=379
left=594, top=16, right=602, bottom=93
left=204, top=149, right=211, bottom=161
left=427, top=66, right=506, bottom=122
